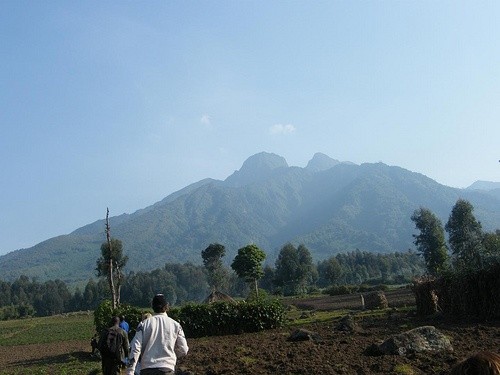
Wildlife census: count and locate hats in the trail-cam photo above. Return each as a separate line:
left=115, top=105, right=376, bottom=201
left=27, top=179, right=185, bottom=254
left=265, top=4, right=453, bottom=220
left=153, top=294, right=168, bottom=306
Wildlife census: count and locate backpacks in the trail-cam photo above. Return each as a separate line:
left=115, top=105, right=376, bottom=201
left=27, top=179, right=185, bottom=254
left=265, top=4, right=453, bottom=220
left=102, top=328, right=124, bottom=353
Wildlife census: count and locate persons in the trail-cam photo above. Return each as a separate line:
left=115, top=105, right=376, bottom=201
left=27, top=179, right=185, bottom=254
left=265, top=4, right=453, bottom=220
left=97, top=315, right=129, bottom=375
left=128, top=293, right=189, bottom=375
left=90, top=334, right=98, bottom=355
left=142, top=312, right=152, bottom=320
